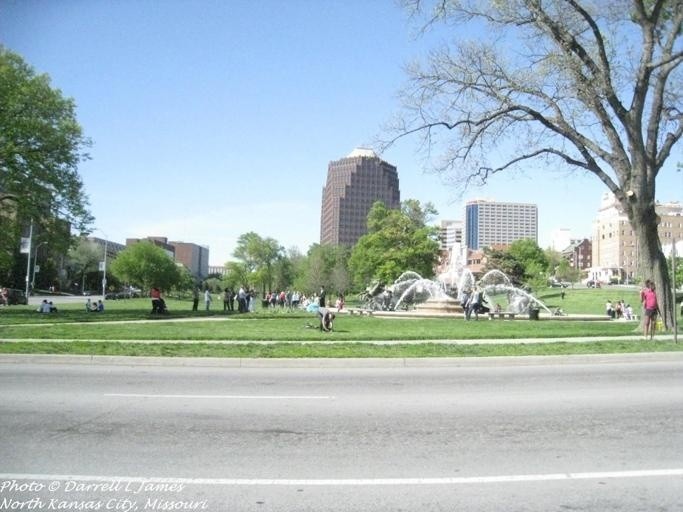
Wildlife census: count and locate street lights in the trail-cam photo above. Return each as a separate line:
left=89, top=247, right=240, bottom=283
left=32, top=242, right=48, bottom=289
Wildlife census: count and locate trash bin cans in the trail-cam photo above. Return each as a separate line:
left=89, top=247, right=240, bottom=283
left=530, top=309, right=539, bottom=320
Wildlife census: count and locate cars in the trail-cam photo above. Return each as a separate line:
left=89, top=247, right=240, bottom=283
left=2, top=287, right=27, bottom=306
left=106, top=289, right=129, bottom=300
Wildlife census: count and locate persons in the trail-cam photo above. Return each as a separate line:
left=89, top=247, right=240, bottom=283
left=192, top=287, right=199, bottom=312
left=223, top=286, right=320, bottom=313
left=336, top=293, right=345, bottom=312
left=40, top=300, right=48, bottom=312
left=88, top=302, right=97, bottom=311
left=86, top=298, right=92, bottom=312
left=317, top=308, right=335, bottom=334
left=606, top=299, right=632, bottom=320
left=561, top=289, right=565, bottom=299
left=318, top=285, right=326, bottom=307
left=462, top=287, right=502, bottom=321
left=150, top=287, right=160, bottom=314
left=0, top=286, right=9, bottom=307
left=43, top=301, right=57, bottom=313
left=640, top=280, right=660, bottom=342
left=51, top=285, right=54, bottom=292
left=97, top=300, right=104, bottom=311
left=204, top=288, right=213, bottom=311
left=48, top=286, right=51, bottom=290
